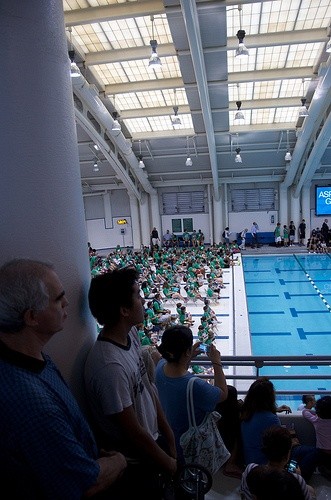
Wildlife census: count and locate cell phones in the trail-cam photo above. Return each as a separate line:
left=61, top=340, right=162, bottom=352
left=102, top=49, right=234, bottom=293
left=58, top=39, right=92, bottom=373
left=288, top=459, right=298, bottom=473
left=197, top=343, right=211, bottom=352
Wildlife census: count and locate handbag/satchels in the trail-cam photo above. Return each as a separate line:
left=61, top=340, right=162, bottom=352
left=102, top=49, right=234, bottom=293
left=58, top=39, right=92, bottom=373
left=222, top=231, right=225, bottom=238
left=177, top=375, right=231, bottom=477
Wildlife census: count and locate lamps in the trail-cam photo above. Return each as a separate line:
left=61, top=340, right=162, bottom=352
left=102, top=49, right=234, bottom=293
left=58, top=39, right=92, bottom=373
left=284, top=130, right=292, bottom=160
left=147, top=15, right=162, bottom=67
left=68, top=26, right=81, bottom=77
left=172, top=90, right=181, bottom=126
left=235, top=3, right=249, bottom=57
left=186, top=137, right=192, bottom=167
left=93, top=158, right=99, bottom=172
left=235, top=133, right=242, bottom=163
left=138, top=139, right=145, bottom=169
left=111, top=95, right=122, bottom=131
left=234, top=82, right=245, bottom=120
left=299, top=77, right=310, bottom=117
left=326, top=26, right=331, bottom=53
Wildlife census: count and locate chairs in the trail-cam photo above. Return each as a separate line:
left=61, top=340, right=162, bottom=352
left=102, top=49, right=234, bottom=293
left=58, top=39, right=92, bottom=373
left=140, top=258, right=223, bottom=377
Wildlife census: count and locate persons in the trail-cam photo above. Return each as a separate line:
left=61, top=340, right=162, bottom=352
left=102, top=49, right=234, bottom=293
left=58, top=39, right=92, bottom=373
left=238, top=229, right=248, bottom=250
left=250, top=222, right=261, bottom=249
left=283, top=225, right=289, bottom=247
left=152, top=326, right=331, bottom=500
left=307, top=219, right=331, bottom=254
left=289, top=221, right=296, bottom=247
left=275, top=223, right=282, bottom=247
left=88, top=226, right=240, bottom=383
left=0, top=258, right=126, bottom=500
left=80, top=267, right=178, bottom=500
left=298, top=219, right=306, bottom=246
